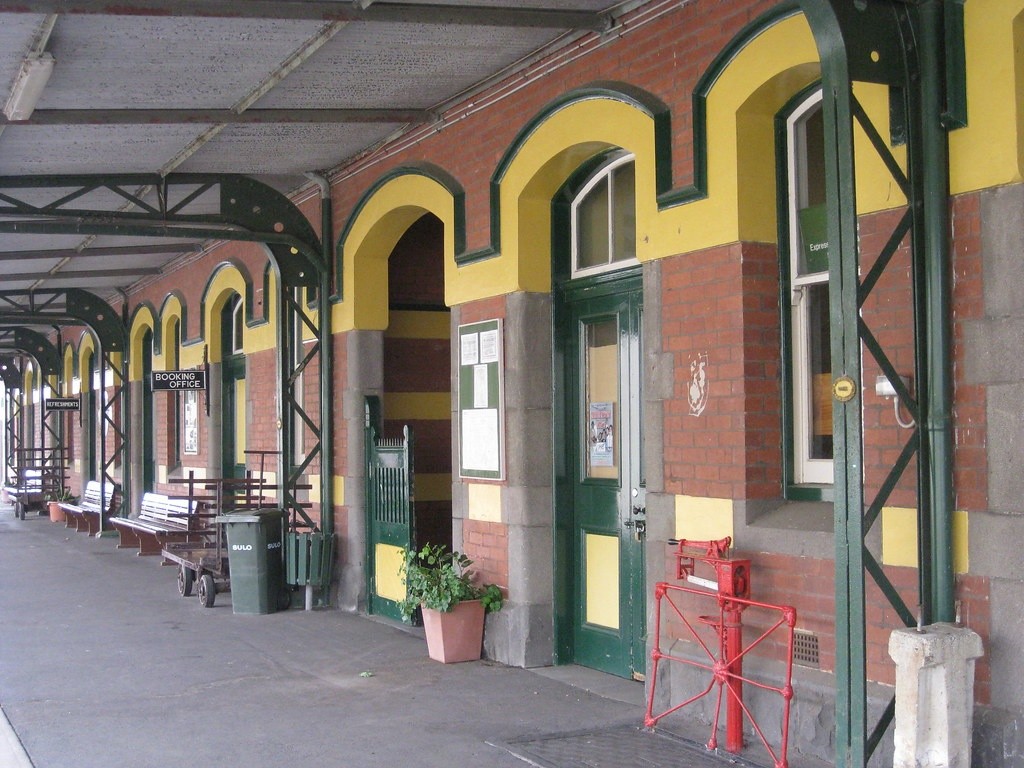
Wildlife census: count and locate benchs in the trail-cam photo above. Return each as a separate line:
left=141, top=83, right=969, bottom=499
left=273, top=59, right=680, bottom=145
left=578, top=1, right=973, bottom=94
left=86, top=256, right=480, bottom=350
left=59, top=480, right=120, bottom=537
left=110, top=493, right=202, bottom=565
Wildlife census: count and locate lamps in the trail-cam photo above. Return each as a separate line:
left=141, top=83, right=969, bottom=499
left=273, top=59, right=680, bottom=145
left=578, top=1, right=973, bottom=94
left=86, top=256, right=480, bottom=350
left=4, top=54, right=56, bottom=121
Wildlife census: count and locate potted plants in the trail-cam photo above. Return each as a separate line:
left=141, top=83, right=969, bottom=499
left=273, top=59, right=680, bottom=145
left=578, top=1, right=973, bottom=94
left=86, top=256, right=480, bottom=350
left=394, top=542, right=502, bottom=665
left=44, top=484, right=81, bottom=522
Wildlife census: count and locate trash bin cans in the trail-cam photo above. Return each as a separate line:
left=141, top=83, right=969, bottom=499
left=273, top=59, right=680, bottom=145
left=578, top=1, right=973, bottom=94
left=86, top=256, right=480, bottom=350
left=284, top=533, right=336, bottom=587
left=215, top=508, right=291, bottom=614
